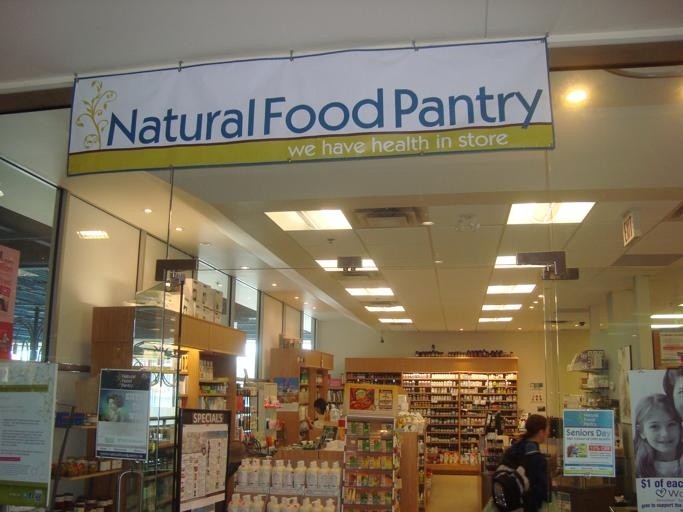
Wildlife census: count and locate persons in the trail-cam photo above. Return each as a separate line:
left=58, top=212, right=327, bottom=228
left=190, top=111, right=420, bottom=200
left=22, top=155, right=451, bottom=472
left=485, top=402, right=502, bottom=434
left=633, top=393, right=683, bottom=478
left=102, top=392, right=129, bottom=423
left=314, top=395, right=327, bottom=420
left=662, top=368, right=683, bottom=421
left=492, top=414, right=552, bottom=512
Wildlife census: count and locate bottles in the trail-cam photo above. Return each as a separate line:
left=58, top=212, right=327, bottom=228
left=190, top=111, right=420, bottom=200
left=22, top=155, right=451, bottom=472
left=248, top=458, right=259, bottom=486
left=291, top=496, right=300, bottom=512
left=323, top=498, right=337, bottom=511
left=294, top=461, right=306, bottom=488
left=237, top=459, right=248, bottom=486
left=311, top=499, right=323, bottom=512
left=266, top=495, right=280, bottom=512
left=284, top=460, right=294, bottom=488
left=199, top=360, right=214, bottom=379
left=318, top=462, right=329, bottom=488
left=251, top=495, right=263, bottom=512
left=306, top=461, right=318, bottom=488
left=300, top=497, right=311, bottom=512
left=272, top=460, right=284, bottom=487
left=329, top=462, right=341, bottom=488
left=52, top=457, right=99, bottom=476
left=54, top=492, right=115, bottom=512
left=260, top=460, right=270, bottom=486
left=240, top=495, right=251, bottom=512
left=227, top=495, right=241, bottom=512
left=280, top=497, right=290, bottom=512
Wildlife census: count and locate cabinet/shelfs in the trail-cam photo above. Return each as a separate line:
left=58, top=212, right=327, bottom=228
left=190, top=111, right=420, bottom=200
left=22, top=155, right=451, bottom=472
left=345, top=357, right=518, bottom=462
left=566, top=350, right=611, bottom=409
left=399, top=432, right=426, bottom=512
left=49, top=403, right=125, bottom=512
left=552, top=477, right=617, bottom=512
left=327, top=386, right=344, bottom=409
left=270, top=349, right=334, bottom=442
left=226, top=449, right=343, bottom=512
left=84, top=306, right=246, bottom=512
left=235, top=376, right=278, bottom=448
left=341, top=414, right=398, bottom=512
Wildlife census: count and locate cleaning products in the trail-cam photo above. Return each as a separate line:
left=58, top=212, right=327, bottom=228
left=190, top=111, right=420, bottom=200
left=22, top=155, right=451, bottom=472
left=200, top=396, right=227, bottom=411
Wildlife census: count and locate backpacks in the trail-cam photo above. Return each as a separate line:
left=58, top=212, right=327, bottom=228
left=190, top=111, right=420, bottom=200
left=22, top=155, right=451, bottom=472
left=491, top=460, right=530, bottom=512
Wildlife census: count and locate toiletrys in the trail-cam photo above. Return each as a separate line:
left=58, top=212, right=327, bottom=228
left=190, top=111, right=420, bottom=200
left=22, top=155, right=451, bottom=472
left=299, top=370, right=310, bottom=419
left=233, top=456, right=343, bottom=494
left=226, top=494, right=337, bottom=512
